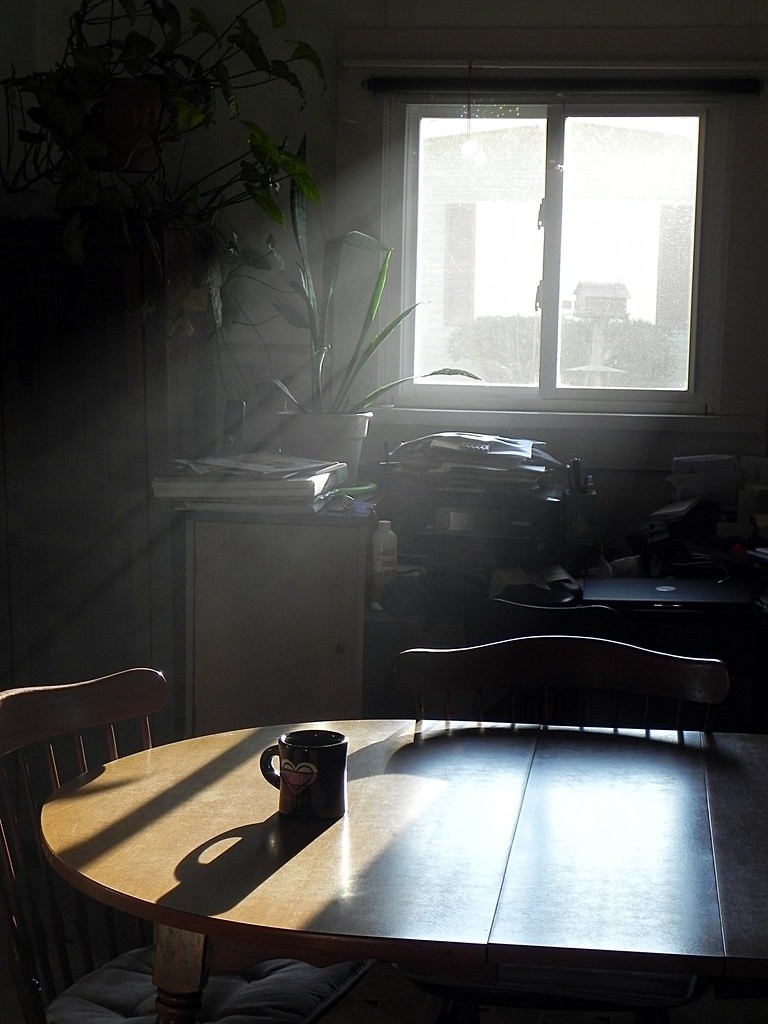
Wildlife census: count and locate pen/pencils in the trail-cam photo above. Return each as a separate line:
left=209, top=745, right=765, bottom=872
left=564, top=458, right=594, bottom=493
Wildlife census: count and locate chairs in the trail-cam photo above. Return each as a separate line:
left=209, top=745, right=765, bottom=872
left=402, top=596, right=730, bottom=1024
left=0, top=668, right=377, bottom=1024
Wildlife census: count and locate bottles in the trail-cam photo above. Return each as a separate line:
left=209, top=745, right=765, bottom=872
left=371, top=520, right=397, bottom=611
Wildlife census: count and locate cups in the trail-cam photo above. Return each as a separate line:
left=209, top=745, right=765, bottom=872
left=260, top=729, right=348, bottom=820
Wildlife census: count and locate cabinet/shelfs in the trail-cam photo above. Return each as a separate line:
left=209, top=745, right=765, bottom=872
left=0, top=219, right=232, bottom=839
left=173, top=492, right=389, bottom=737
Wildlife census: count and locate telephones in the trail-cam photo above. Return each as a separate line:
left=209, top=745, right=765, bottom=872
left=213, top=399, right=246, bottom=458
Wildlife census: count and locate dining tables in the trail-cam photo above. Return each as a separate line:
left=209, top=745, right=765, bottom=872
left=37, top=721, right=768, bottom=1024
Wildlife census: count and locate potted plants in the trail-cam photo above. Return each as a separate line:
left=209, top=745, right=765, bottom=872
left=0, top=0, right=332, bottom=342
left=238, top=135, right=483, bottom=488
